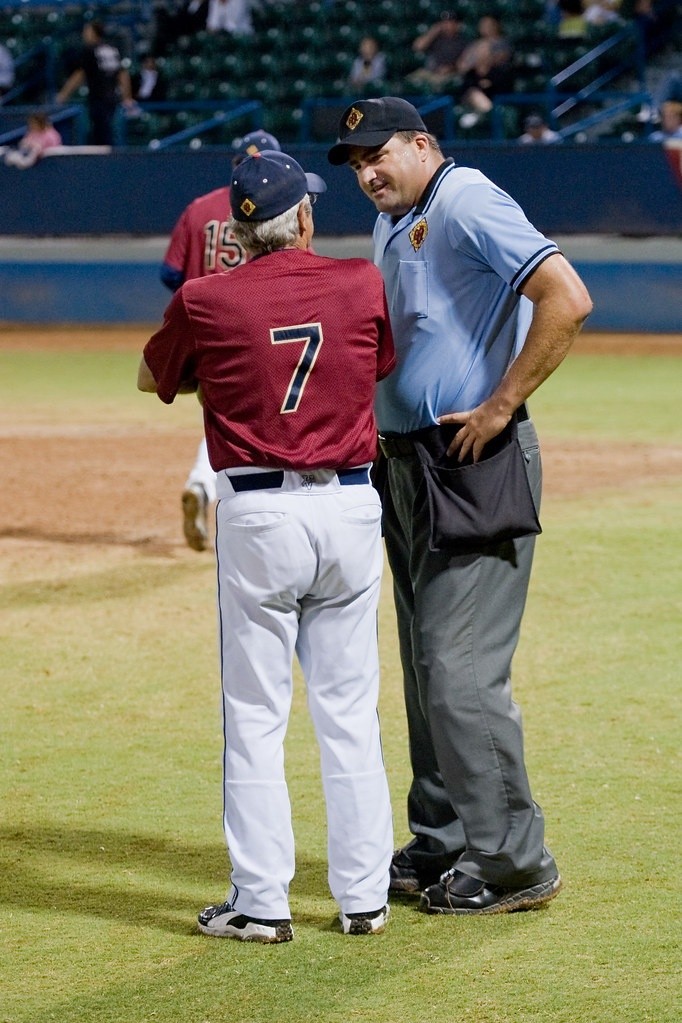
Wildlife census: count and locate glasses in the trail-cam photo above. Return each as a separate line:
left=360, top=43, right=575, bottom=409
left=306, top=193, right=320, bottom=205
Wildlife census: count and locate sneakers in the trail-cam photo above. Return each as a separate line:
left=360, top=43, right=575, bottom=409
left=389, top=835, right=466, bottom=896
left=418, top=869, right=563, bottom=915
left=197, top=901, right=294, bottom=944
left=338, top=902, right=390, bottom=934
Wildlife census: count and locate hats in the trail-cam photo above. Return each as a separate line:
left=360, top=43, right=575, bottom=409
left=235, top=130, right=281, bottom=156
left=230, top=150, right=328, bottom=221
left=328, top=97, right=429, bottom=166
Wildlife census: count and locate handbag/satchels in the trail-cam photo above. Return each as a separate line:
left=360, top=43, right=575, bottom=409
left=412, top=412, right=542, bottom=553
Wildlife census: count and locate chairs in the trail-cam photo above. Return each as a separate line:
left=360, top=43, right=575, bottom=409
left=0, top=0, right=682, bottom=143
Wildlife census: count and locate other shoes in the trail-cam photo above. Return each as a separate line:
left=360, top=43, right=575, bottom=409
left=182, top=482, right=209, bottom=551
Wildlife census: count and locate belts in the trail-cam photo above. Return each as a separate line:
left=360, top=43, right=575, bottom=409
left=227, top=468, right=369, bottom=492
left=379, top=402, right=528, bottom=458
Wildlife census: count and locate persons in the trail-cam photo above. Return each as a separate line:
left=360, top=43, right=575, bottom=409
left=0, top=0, right=256, bottom=168
left=158, top=129, right=280, bottom=552
left=138, top=149, right=396, bottom=944
left=326, top=99, right=592, bottom=915
left=347, top=0, right=682, bottom=142
